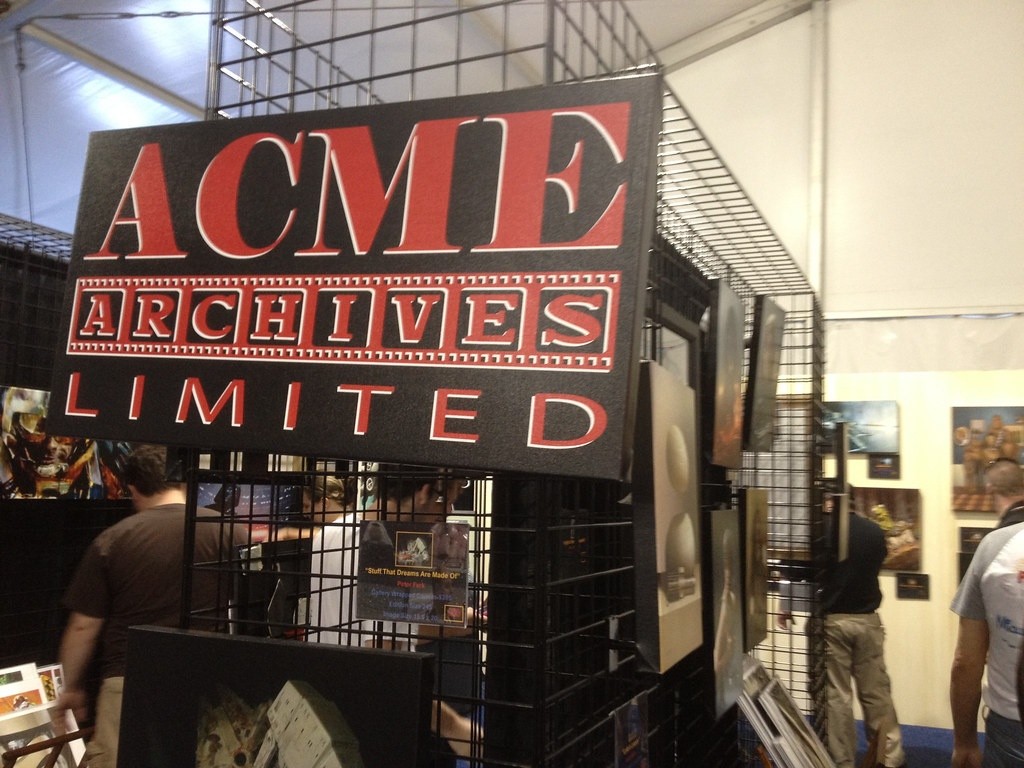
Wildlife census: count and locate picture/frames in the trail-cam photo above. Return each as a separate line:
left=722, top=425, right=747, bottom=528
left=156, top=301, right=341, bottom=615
left=758, top=675, right=837, bottom=768
left=739, top=663, right=787, bottom=768
left=773, top=730, right=804, bottom=768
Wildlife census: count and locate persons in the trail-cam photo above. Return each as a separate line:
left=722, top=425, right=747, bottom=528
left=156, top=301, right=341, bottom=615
left=948, top=522, right=1024, bottom=768
left=983, top=457, right=1024, bottom=530
left=50, top=445, right=256, bottom=768
left=263, top=463, right=488, bottom=768
left=776, top=478, right=906, bottom=768
